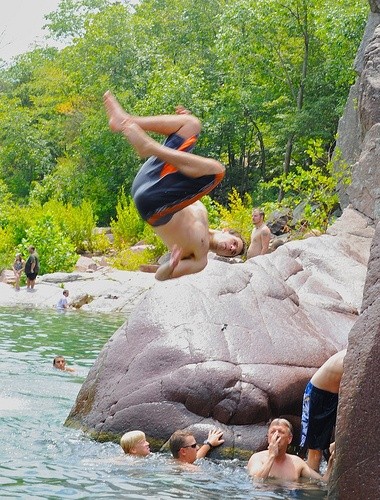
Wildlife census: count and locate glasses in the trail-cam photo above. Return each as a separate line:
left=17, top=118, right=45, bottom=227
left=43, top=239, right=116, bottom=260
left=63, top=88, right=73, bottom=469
left=183, top=443, right=196, bottom=448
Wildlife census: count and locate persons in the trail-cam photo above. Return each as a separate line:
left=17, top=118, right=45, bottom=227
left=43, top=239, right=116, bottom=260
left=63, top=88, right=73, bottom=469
left=53, top=356, right=76, bottom=372
left=300, top=219, right=321, bottom=239
left=25, top=246, right=39, bottom=289
left=170, top=429, right=225, bottom=463
left=12, top=253, right=25, bottom=289
left=299, top=349, right=347, bottom=472
left=57, top=290, right=69, bottom=310
left=120, top=430, right=151, bottom=457
left=247, top=418, right=335, bottom=489
left=247, top=209, right=270, bottom=259
left=103, top=90, right=246, bottom=281
